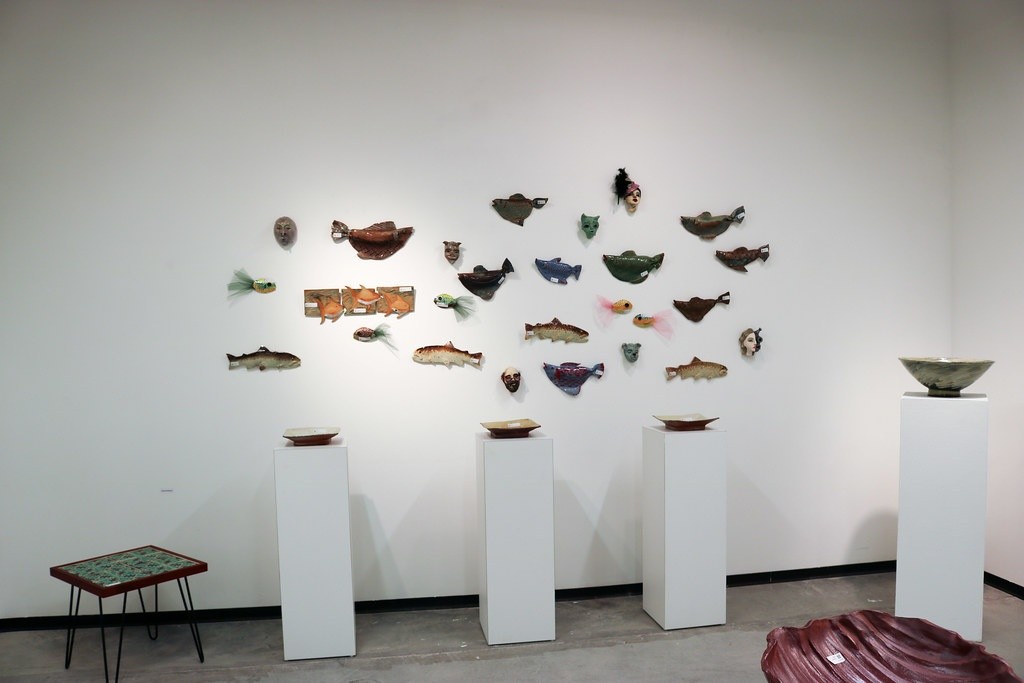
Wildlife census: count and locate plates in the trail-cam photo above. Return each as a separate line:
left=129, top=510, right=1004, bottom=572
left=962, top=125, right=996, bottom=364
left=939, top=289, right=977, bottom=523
left=282, top=426, right=340, bottom=446
left=480, top=418, right=541, bottom=439
left=653, top=413, right=719, bottom=431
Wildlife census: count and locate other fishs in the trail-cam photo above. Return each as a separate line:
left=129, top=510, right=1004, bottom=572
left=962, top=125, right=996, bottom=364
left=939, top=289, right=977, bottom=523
left=224, top=193, right=770, bottom=395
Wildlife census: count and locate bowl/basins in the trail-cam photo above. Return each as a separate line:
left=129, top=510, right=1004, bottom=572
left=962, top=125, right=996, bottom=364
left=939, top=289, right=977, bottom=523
left=898, top=356, right=995, bottom=398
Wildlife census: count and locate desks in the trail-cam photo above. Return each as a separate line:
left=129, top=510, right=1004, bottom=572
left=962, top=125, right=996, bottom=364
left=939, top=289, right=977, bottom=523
left=49, top=545, right=209, bottom=683
